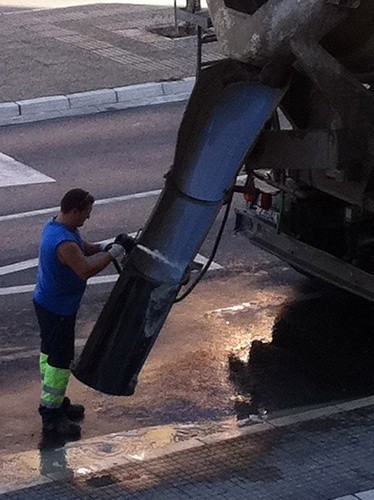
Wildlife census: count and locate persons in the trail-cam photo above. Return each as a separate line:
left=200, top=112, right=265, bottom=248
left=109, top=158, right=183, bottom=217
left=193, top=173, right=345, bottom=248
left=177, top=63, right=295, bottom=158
left=33, top=186, right=131, bottom=440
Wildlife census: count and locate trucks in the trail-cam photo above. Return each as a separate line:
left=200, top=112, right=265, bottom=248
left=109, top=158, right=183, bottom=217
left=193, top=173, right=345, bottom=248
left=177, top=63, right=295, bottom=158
left=178, top=0, right=374, bottom=303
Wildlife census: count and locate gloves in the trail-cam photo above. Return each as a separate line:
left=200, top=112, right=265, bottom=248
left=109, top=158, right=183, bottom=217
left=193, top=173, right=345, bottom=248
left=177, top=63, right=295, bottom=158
left=105, top=233, right=137, bottom=262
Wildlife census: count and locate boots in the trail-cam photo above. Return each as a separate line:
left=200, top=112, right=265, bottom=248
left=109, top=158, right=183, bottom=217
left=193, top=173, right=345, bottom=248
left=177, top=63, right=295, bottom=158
left=39, top=407, right=82, bottom=436
left=62, top=397, right=84, bottom=417
